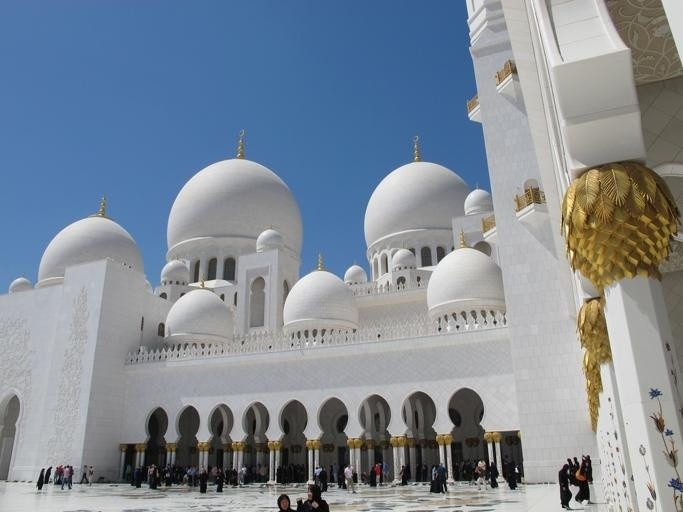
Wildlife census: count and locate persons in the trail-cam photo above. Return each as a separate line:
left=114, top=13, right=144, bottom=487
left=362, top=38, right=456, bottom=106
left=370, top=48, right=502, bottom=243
left=296, top=484, right=329, bottom=512
left=399, top=463, right=449, bottom=494
left=276, top=493, right=298, bottom=512
left=38, top=465, right=96, bottom=490
left=452, top=456, right=521, bottom=490
left=125, top=462, right=390, bottom=494
left=558, top=454, right=594, bottom=511
